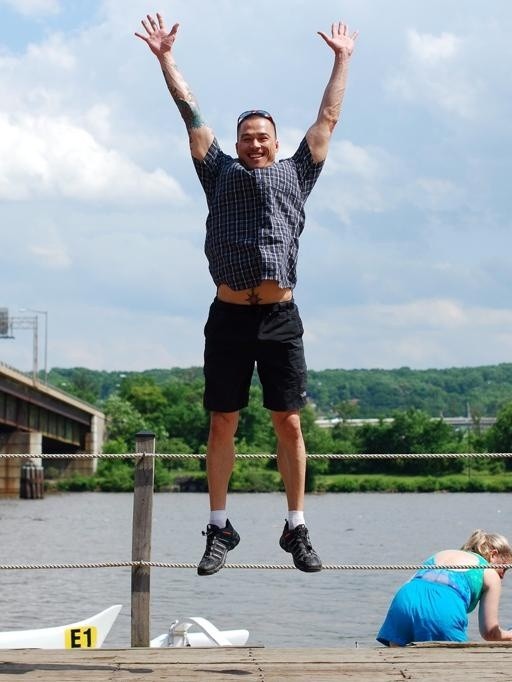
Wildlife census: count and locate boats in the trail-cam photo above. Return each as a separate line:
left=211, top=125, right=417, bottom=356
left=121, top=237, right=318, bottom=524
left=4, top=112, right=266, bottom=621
left=0, top=603, right=125, bottom=651
left=148, top=629, right=250, bottom=650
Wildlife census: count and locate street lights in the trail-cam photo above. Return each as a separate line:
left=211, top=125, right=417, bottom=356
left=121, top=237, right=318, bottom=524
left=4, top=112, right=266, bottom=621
left=16, top=307, right=48, bottom=385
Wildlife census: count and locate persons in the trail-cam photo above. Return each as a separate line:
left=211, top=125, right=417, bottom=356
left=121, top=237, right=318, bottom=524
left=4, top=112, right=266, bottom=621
left=376, top=527, right=511, bottom=647
left=134, top=9, right=361, bottom=575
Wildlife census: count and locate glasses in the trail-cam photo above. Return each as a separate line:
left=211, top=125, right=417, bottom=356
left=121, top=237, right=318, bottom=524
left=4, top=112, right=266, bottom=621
left=237, top=109, right=271, bottom=124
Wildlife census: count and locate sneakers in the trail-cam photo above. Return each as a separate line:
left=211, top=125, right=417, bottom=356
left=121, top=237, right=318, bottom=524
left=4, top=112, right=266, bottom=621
left=279, top=516, right=322, bottom=572
left=197, top=518, right=241, bottom=576
